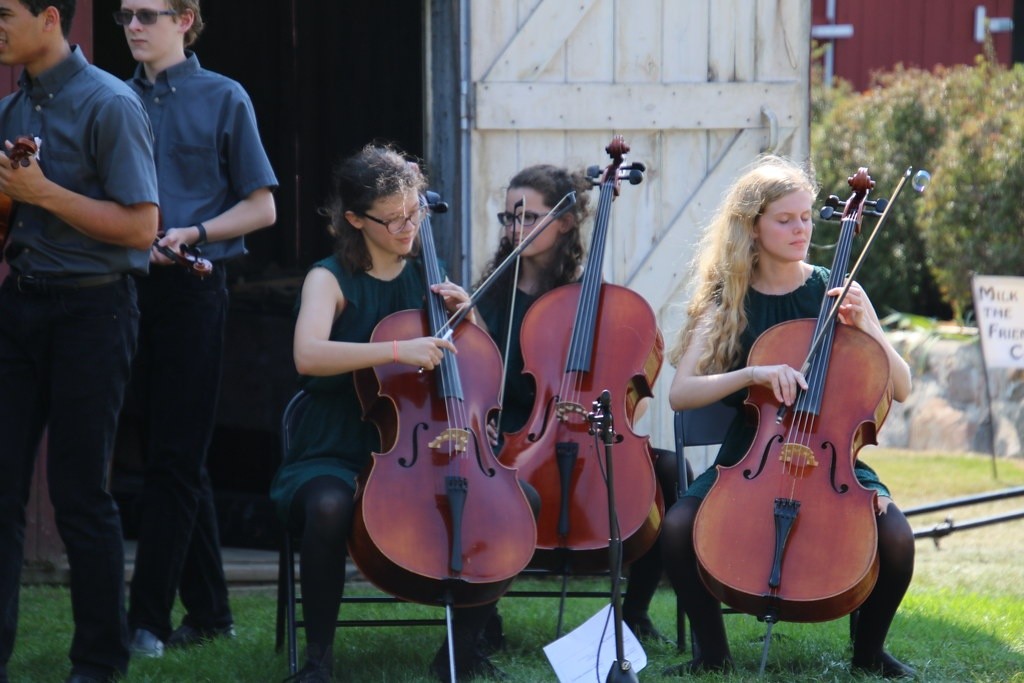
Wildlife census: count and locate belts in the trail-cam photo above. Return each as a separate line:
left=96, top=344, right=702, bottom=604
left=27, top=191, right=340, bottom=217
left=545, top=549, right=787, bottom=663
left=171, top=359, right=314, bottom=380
left=7, top=270, right=132, bottom=293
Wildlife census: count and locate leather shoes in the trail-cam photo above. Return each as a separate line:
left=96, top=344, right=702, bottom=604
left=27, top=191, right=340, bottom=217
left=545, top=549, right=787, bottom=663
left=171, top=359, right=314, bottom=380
left=125, top=628, right=165, bottom=661
left=164, top=622, right=230, bottom=650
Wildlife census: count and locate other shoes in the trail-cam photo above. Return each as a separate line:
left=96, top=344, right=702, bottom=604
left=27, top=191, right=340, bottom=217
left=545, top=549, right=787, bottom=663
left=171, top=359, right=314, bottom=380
left=65, top=674, right=98, bottom=683
left=850, top=652, right=923, bottom=682
left=621, top=606, right=677, bottom=651
left=661, top=655, right=739, bottom=681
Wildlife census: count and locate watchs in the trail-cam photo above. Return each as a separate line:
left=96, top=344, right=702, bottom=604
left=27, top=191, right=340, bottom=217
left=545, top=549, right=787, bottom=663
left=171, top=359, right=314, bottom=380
left=188, top=223, right=208, bottom=247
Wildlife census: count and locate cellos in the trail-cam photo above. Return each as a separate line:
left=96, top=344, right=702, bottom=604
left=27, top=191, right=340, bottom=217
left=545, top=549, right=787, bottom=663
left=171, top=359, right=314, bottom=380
left=496, top=135, right=665, bottom=638
left=352, top=185, right=538, bottom=683
left=693, top=167, right=896, bottom=679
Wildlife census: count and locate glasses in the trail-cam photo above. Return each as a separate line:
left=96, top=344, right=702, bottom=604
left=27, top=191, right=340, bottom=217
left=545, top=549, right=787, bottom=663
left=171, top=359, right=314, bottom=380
left=352, top=198, right=427, bottom=235
left=112, top=9, right=181, bottom=26
left=497, top=212, right=558, bottom=227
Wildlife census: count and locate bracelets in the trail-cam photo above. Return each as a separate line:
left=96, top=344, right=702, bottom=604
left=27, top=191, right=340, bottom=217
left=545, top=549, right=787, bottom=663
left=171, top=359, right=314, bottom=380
left=752, top=366, right=757, bottom=377
left=392, top=340, right=398, bottom=364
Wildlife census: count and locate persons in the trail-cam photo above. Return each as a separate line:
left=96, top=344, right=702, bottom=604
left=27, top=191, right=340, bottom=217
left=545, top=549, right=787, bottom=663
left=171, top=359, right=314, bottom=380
left=270, top=143, right=501, bottom=683
left=0, top=0, right=160, bottom=683
left=472, top=165, right=671, bottom=657
left=96, top=0, right=278, bottom=655
left=659, top=156, right=921, bottom=683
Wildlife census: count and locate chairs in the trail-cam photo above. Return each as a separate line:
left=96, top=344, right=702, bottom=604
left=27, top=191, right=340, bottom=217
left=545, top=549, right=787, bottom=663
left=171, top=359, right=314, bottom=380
left=675, top=400, right=746, bottom=667
left=274, top=388, right=445, bottom=683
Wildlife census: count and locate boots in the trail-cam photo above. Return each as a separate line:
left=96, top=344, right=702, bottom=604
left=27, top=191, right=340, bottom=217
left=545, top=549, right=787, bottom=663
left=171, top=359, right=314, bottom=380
left=283, top=641, right=334, bottom=683
left=429, top=606, right=506, bottom=683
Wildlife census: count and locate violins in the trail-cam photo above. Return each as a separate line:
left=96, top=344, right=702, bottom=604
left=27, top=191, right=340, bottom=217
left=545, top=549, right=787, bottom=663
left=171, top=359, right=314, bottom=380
left=152, top=233, right=216, bottom=281
left=0, top=129, right=39, bottom=256
